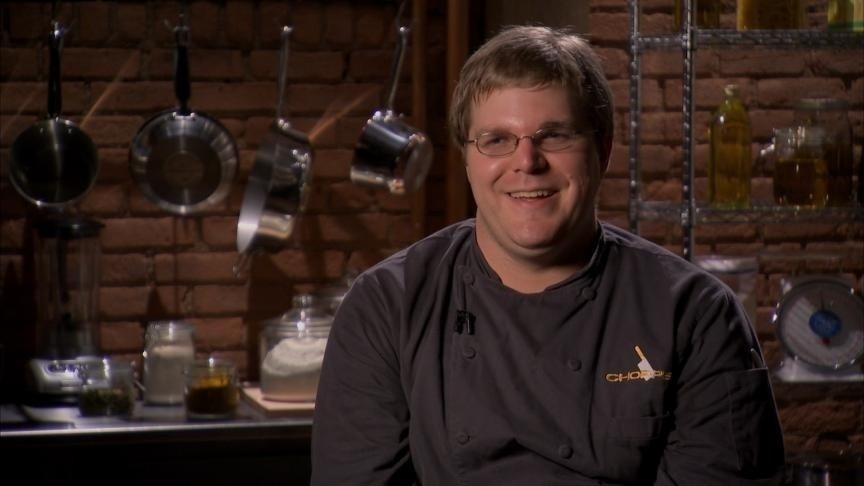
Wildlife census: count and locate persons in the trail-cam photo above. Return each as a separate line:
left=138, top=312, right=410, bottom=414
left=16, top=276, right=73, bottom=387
left=310, top=25, right=791, bottom=484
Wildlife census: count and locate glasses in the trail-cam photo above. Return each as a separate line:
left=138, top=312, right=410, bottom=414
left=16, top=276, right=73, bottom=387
left=465, top=127, right=585, bottom=156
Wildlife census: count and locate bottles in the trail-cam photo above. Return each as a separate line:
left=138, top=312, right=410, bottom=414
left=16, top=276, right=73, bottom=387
left=140, top=286, right=362, bottom=406
left=706, top=83, right=855, bottom=211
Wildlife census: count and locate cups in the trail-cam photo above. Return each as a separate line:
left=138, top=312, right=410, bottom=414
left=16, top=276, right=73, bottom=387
left=77, top=358, right=137, bottom=425
left=184, top=358, right=242, bottom=422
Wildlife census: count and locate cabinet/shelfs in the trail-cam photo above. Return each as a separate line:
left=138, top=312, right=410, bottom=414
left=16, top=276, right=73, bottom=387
left=630, top=1, right=862, bottom=265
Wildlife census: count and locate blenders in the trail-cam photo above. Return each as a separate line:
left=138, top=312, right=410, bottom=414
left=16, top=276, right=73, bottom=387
left=20, top=220, right=106, bottom=423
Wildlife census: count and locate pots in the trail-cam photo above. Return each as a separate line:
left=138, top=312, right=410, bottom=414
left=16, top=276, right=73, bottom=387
left=127, top=24, right=238, bottom=218
left=238, top=26, right=315, bottom=256
left=342, top=15, right=433, bottom=203
left=7, top=19, right=99, bottom=215
